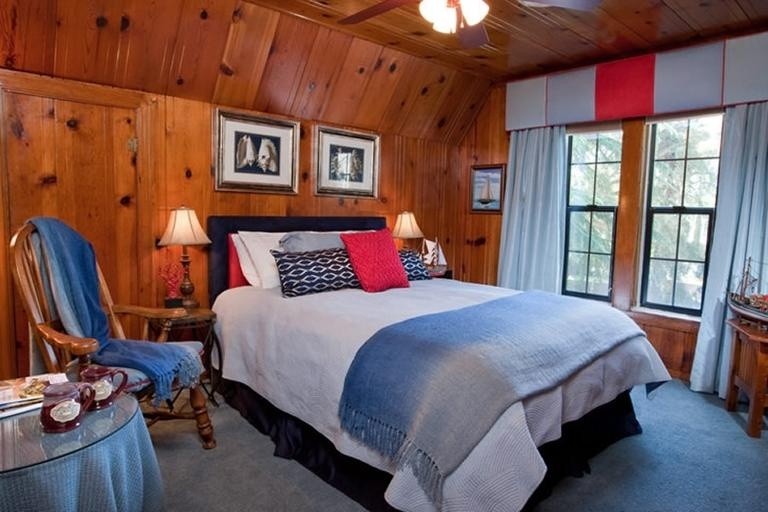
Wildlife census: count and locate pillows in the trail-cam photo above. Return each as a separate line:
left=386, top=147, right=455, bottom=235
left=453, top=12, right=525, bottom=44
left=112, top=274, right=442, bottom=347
left=281, top=232, right=344, bottom=253
left=237, top=227, right=293, bottom=289
left=340, top=229, right=409, bottom=293
left=400, top=251, right=431, bottom=281
left=230, top=234, right=261, bottom=286
left=268, top=248, right=362, bottom=298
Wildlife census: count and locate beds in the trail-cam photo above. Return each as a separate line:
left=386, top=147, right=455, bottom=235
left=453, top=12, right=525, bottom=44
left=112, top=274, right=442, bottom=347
left=208, top=213, right=671, bottom=511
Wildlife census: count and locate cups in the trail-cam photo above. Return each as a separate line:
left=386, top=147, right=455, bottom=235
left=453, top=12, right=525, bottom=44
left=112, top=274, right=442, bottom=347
left=78, top=365, right=128, bottom=412
left=40, top=381, right=96, bottom=434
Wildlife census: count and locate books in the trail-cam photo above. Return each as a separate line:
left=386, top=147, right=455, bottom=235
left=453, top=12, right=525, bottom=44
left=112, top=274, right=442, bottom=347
left=0, top=371, right=71, bottom=420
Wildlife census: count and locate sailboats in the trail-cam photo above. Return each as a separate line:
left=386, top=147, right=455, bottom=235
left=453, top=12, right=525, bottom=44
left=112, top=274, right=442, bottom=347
left=476, top=175, right=496, bottom=205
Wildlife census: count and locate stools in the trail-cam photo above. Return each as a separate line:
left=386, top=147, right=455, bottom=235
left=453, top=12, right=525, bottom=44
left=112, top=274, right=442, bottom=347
left=151, top=308, right=221, bottom=413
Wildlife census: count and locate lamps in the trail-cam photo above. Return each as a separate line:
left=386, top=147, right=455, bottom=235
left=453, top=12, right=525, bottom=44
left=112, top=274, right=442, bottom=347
left=419, top=0, right=490, bottom=34
left=392, top=211, right=423, bottom=249
left=156, top=203, right=211, bottom=309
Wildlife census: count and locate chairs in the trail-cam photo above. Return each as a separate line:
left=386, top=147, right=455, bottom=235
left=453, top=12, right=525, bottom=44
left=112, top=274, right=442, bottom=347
left=7, top=217, right=218, bottom=450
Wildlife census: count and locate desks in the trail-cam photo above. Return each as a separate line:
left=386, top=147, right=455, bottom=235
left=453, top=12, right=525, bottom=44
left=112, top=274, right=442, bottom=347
left=0, top=364, right=161, bottom=512
left=721, top=320, right=768, bottom=439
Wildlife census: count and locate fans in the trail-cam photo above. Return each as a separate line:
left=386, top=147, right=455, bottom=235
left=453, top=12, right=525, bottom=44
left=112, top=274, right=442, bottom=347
left=336, top=0, right=600, bottom=49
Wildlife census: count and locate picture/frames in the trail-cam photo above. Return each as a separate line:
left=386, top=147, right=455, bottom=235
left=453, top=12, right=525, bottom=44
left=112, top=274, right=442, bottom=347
left=468, top=161, right=508, bottom=212
left=212, top=101, right=301, bottom=194
left=310, top=125, right=381, bottom=198
